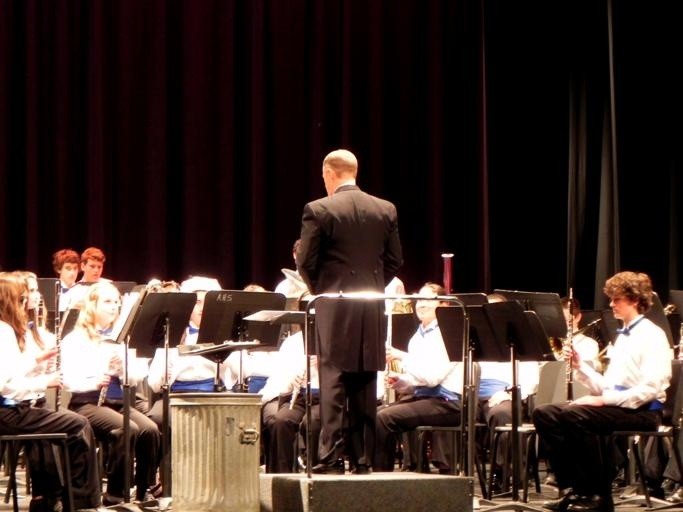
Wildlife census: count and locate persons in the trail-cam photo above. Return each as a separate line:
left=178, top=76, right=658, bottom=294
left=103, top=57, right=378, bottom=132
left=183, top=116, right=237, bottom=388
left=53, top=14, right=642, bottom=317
left=367, top=269, right=674, bottom=511
left=294, top=145, right=405, bottom=474
left=0, top=245, right=326, bottom=512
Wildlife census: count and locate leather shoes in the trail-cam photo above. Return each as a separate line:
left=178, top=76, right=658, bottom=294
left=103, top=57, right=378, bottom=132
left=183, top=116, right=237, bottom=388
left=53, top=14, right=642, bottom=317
left=542, top=493, right=590, bottom=511
left=566, top=495, right=615, bottom=512
left=312, top=460, right=345, bottom=475
left=352, top=463, right=373, bottom=474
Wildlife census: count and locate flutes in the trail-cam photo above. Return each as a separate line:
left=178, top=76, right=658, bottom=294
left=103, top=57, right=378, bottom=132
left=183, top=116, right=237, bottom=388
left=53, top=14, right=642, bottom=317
left=54, top=281, right=61, bottom=412
left=288, top=367, right=306, bottom=412
left=96, top=384, right=110, bottom=407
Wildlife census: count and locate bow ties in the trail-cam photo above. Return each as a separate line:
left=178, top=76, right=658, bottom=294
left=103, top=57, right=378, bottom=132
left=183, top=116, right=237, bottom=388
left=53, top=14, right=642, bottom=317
left=615, top=327, right=629, bottom=336
left=188, top=327, right=199, bottom=334
left=98, top=329, right=112, bottom=336
